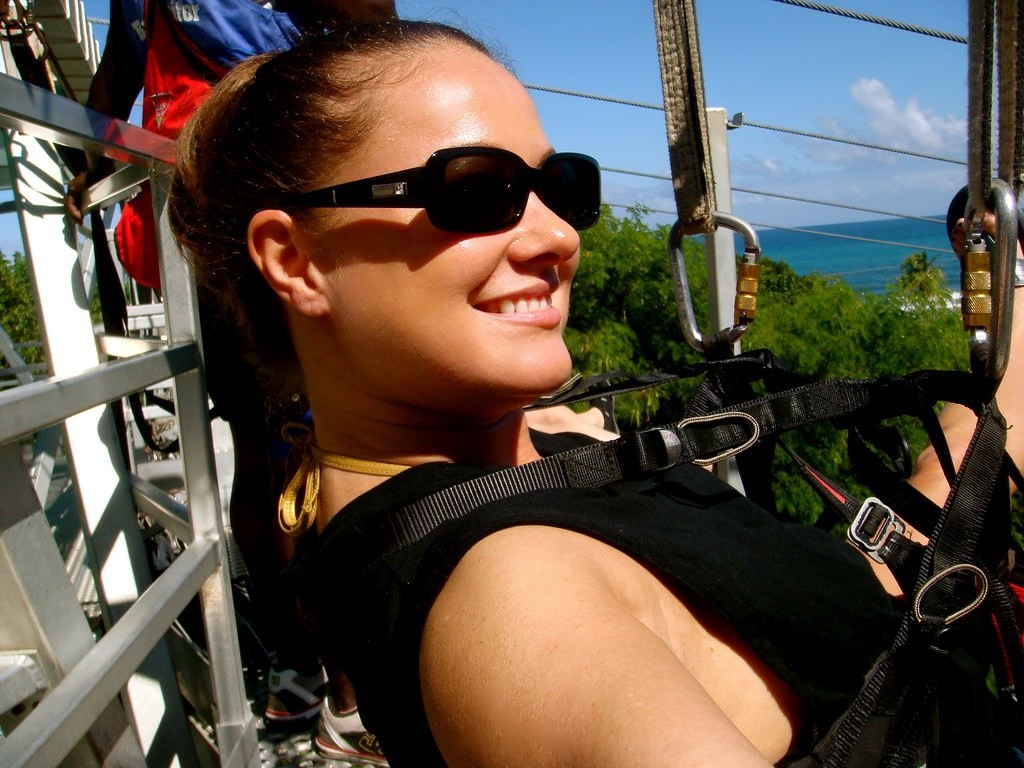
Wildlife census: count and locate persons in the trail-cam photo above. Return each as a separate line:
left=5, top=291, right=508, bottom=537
left=162, top=23, right=1024, bottom=767
left=66, top=1, right=400, bottom=768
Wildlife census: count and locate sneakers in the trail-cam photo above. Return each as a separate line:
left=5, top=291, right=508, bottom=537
left=258, top=662, right=392, bottom=766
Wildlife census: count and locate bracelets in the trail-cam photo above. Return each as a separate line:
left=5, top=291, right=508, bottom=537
left=1015, top=258, right=1024, bottom=287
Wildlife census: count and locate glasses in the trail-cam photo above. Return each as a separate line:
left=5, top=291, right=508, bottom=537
left=280, top=148, right=602, bottom=236
